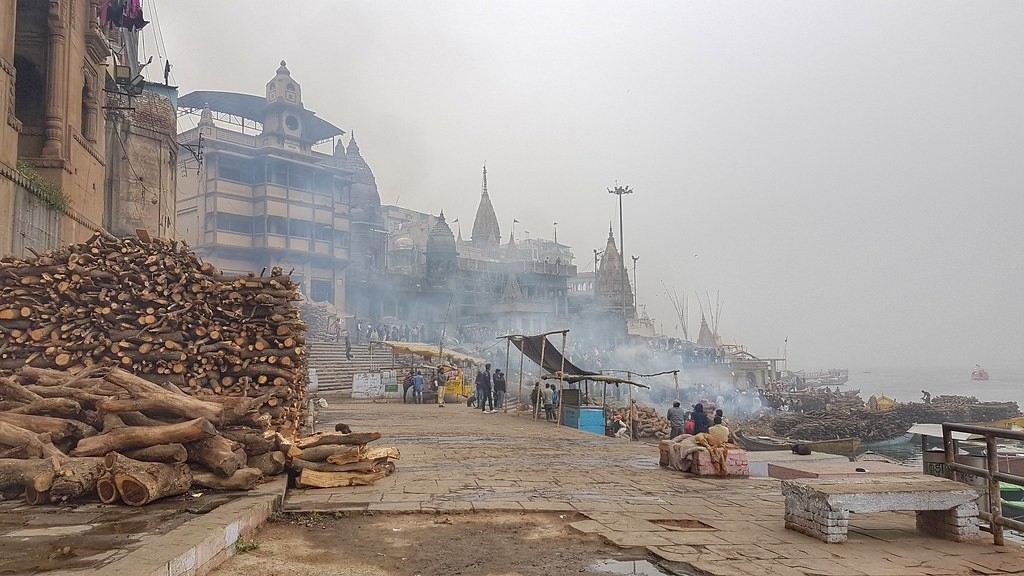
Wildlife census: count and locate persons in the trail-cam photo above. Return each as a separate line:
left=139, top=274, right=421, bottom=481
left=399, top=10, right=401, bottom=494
left=345, top=337, right=355, bottom=362
left=546, top=257, right=548, bottom=264
left=556, top=257, right=561, bottom=264
left=608, top=415, right=626, bottom=437
left=438, top=368, right=447, bottom=407
left=542, top=383, right=559, bottom=419
left=366, top=323, right=425, bottom=348
left=334, top=318, right=341, bottom=342
left=922, top=390, right=931, bottom=403
left=532, top=382, right=543, bottom=419
left=667, top=400, right=734, bottom=443
left=403, top=371, right=424, bottom=404
left=493, top=369, right=505, bottom=408
left=648, top=337, right=841, bottom=416
left=868, top=395, right=879, bottom=410
left=628, top=400, right=639, bottom=441
left=456, top=326, right=601, bottom=371
left=475, top=364, right=499, bottom=414
left=357, top=320, right=363, bottom=341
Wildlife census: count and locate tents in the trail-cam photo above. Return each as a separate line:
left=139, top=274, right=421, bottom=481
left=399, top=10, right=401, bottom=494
left=496, top=330, right=649, bottom=427
left=371, top=340, right=487, bottom=397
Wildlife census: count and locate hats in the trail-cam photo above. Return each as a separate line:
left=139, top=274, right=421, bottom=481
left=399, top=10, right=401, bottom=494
left=673, top=399, right=680, bottom=406
left=632, top=398, right=636, bottom=403
left=616, top=415, right=622, bottom=419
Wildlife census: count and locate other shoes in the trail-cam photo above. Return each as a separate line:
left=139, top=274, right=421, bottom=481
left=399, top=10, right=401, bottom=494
left=347, top=360, right=351, bottom=362
left=482, top=410, right=490, bottom=414
left=439, top=405, right=444, bottom=407
left=353, top=356, right=355, bottom=359
left=491, top=409, right=499, bottom=413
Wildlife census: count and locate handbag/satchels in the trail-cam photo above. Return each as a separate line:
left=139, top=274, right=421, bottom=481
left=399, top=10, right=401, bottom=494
left=686, top=421, right=694, bottom=433
